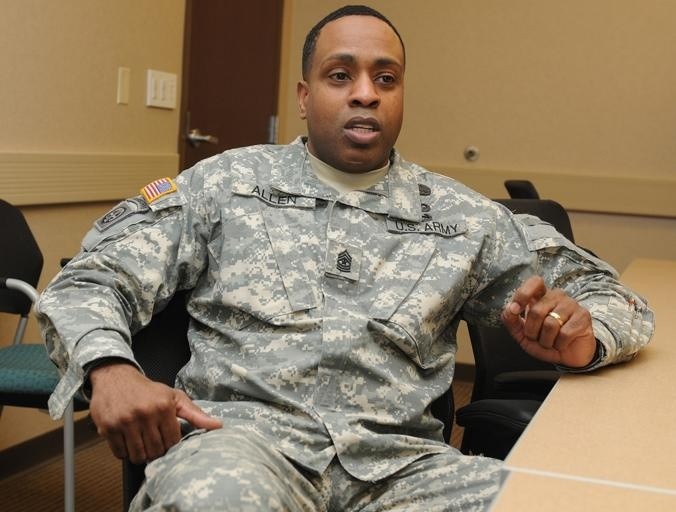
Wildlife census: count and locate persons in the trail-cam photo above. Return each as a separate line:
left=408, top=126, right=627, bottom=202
left=32, top=5, right=655, bottom=512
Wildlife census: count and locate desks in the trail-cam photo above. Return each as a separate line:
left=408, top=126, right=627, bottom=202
left=487, top=257, right=676, bottom=512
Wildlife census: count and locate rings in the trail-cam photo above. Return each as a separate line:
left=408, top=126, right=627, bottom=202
left=547, top=311, right=563, bottom=327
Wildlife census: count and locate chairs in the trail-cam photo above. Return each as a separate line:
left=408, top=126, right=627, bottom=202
left=454, top=198, right=601, bottom=457
left=0, top=198, right=91, bottom=511
